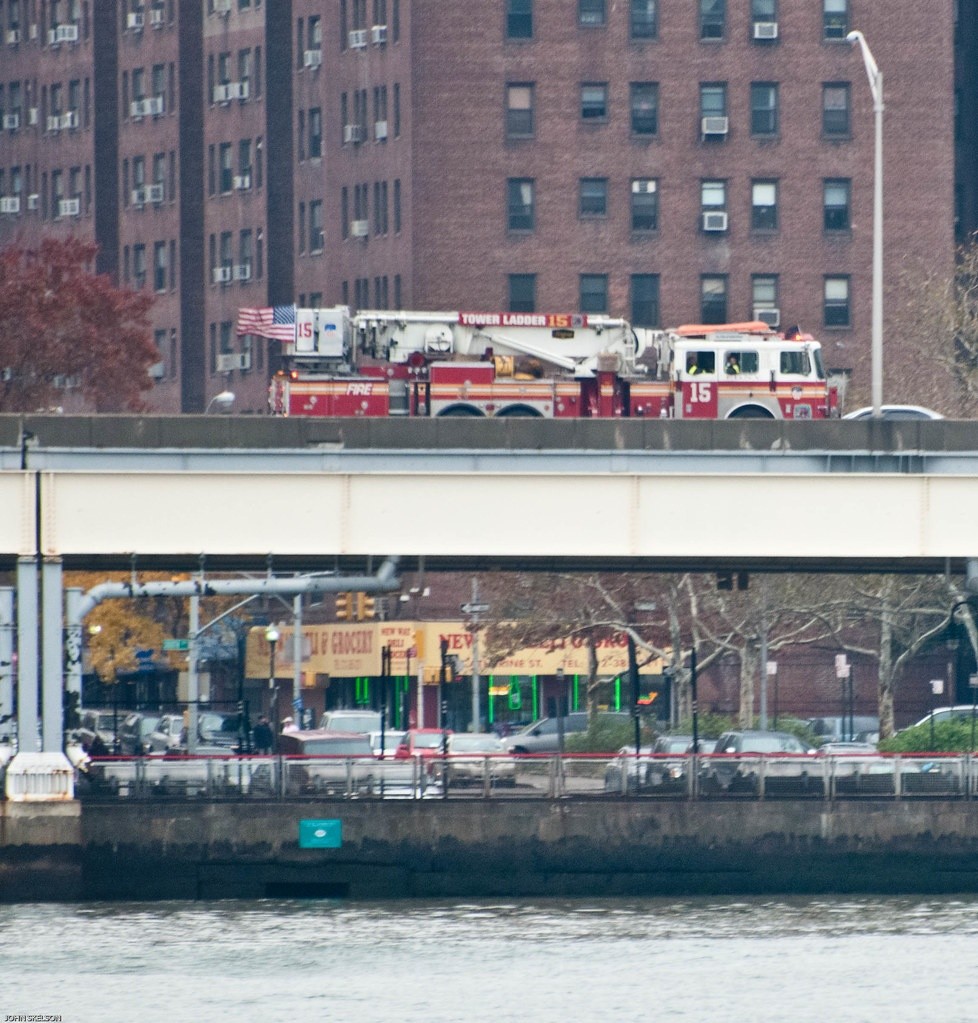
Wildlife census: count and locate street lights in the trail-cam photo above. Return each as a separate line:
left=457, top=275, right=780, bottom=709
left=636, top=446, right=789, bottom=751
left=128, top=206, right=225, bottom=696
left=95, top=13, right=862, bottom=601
left=264, top=622, right=280, bottom=759
left=845, top=29, right=886, bottom=417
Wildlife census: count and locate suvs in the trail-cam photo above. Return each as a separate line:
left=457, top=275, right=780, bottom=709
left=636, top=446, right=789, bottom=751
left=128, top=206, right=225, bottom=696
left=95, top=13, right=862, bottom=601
left=180, top=709, right=259, bottom=755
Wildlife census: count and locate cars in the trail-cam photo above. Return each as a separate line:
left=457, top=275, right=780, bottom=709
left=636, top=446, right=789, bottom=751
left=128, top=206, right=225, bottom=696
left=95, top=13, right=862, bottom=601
left=604, top=746, right=654, bottom=791
left=150, top=714, right=185, bottom=752
left=393, top=728, right=453, bottom=774
left=247, top=710, right=443, bottom=800
left=840, top=405, right=946, bottom=420
left=431, top=731, right=518, bottom=789
left=116, top=712, right=163, bottom=756
left=73, top=708, right=130, bottom=762
left=803, top=704, right=978, bottom=746
left=644, top=730, right=921, bottom=788
left=159, top=747, right=254, bottom=796
left=500, top=712, right=663, bottom=759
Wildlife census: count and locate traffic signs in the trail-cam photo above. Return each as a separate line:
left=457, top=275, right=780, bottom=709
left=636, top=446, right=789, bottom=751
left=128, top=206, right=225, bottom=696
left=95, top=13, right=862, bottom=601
left=459, top=602, right=491, bottom=614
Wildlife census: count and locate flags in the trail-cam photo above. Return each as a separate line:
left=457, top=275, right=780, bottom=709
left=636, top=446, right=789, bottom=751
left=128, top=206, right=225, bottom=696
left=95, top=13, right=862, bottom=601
left=236, top=303, right=297, bottom=343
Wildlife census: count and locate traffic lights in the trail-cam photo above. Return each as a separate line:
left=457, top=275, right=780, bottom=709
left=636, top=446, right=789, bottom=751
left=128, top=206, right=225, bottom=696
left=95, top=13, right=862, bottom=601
left=336, top=592, right=353, bottom=621
left=717, top=572, right=733, bottom=591
left=357, top=592, right=376, bottom=621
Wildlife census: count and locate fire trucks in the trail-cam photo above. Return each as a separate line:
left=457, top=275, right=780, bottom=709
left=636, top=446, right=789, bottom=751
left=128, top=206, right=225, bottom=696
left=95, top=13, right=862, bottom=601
left=268, top=305, right=839, bottom=420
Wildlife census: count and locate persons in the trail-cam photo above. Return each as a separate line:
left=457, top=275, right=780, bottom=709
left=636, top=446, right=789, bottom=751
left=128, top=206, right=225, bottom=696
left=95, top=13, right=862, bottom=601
left=66, top=733, right=109, bottom=769
left=686, top=353, right=740, bottom=375
left=492, top=716, right=512, bottom=737
left=0, top=736, right=12, bottom=768
left=253, top=715, right=299, bottom=756
